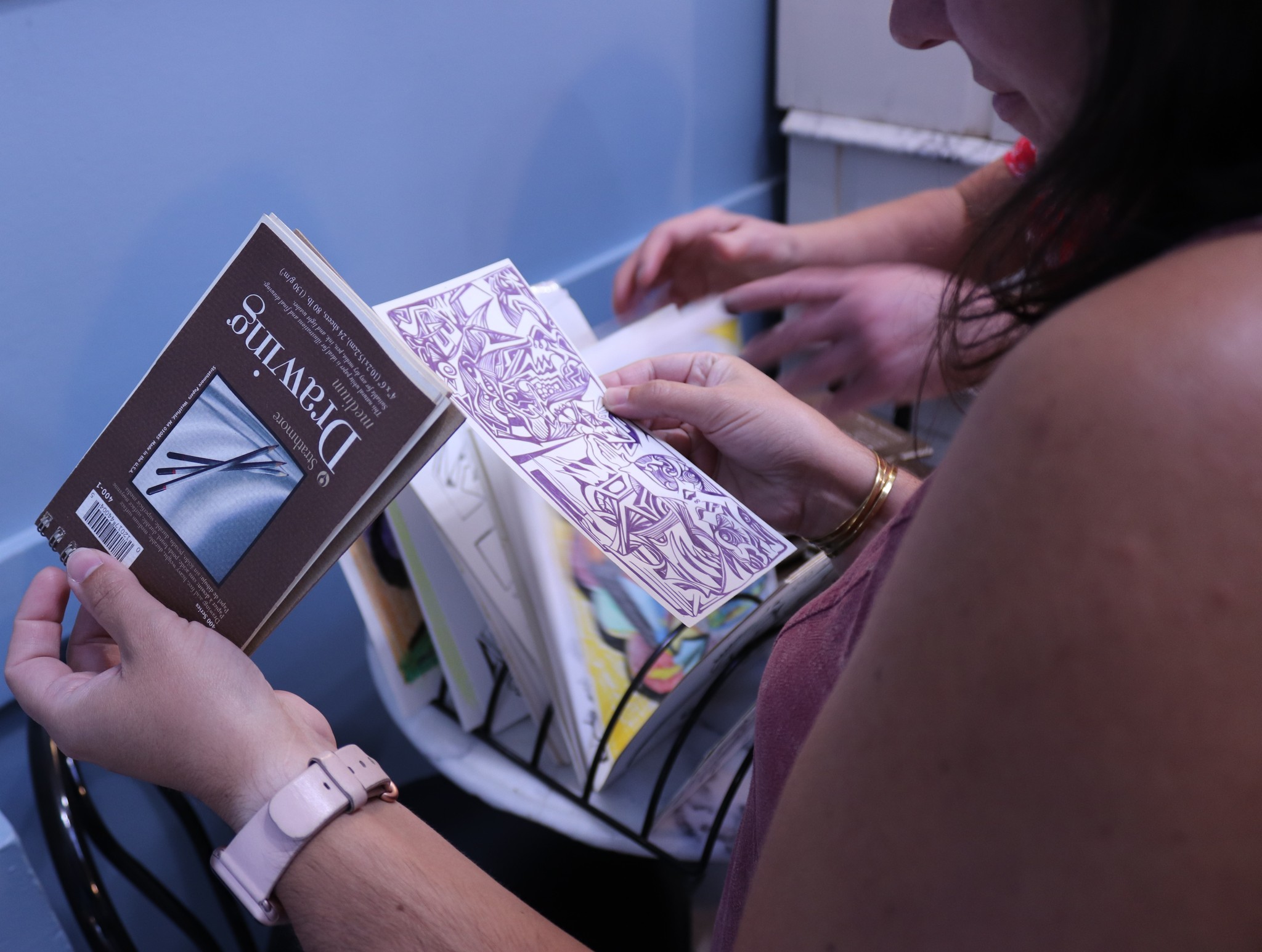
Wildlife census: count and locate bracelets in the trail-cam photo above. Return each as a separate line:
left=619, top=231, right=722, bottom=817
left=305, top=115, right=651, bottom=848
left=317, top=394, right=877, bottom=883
left=805, top=451, right=897, bottom=557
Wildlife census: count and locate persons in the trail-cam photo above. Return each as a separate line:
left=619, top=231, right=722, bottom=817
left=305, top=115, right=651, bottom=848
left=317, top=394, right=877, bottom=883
left=3, top=0, right=1262, bottom=952
left=612, top=134, right=1039, bottom=422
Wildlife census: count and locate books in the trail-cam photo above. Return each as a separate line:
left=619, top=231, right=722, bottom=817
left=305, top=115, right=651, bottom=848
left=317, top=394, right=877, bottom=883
left=35, top=211, right=840, bottom=839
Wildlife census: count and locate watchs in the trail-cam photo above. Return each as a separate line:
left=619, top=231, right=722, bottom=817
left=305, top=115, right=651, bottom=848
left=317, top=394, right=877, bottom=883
left=206, top=744, right=397, bottom=928
